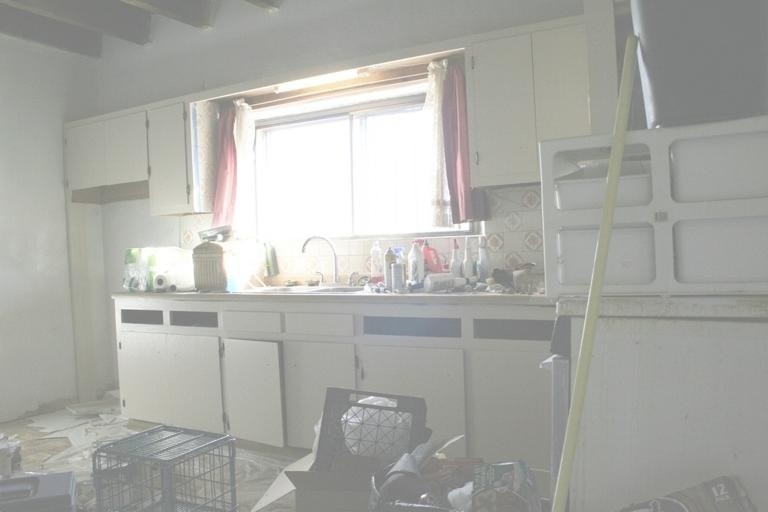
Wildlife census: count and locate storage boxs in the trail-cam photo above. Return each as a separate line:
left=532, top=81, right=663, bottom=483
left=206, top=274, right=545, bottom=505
left=244, top=423, right=448, bottom=512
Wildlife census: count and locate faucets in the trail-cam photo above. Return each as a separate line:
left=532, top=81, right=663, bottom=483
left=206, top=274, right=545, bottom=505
left=302, top=236, right=338, bottom=283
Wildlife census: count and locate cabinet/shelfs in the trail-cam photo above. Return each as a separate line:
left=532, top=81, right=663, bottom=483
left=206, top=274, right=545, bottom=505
left=224, top=307, right=360, bottom=453
left=361, top=307, right=555, bottom=475
left=460, top=14, right=589, bottom=190
left=63, top=112, right=149, bottom=192
left=540, top=116, right=768, bottom=302
left=147, top=101, right=223, bottom=218
left=116, top=301, right=227, bottom=440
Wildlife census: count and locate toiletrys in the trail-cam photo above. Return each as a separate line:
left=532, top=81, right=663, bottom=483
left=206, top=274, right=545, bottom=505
left=462, top=236, right=473, bottom=279
left=477, top=236, right=490, bottom=282
left=449, top=238, right=461, bottom=276
left=368, top=239, right=444, bottom=296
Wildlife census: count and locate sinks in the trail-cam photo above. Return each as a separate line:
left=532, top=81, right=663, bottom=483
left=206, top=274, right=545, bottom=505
left=249, top=286, right=309, bottom=294
left=309, top=287, right=366, bottom=294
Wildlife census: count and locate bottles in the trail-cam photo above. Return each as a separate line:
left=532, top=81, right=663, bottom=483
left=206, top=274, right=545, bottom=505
left=370, top=243, right=488, bottom=293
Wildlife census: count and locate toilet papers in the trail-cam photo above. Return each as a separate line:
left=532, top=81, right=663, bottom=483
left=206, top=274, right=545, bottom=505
left=152, top=273, right=170, bottom=288
